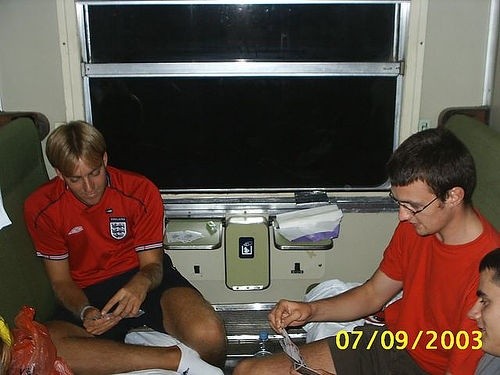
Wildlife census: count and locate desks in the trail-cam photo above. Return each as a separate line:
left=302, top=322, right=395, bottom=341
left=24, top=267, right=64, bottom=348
left=272, top=218, right=334, bottom=250
left=163, top=219, right=222, bottom=250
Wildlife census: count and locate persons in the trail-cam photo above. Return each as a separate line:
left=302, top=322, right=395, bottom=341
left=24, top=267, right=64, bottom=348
left=466, top=247, right=500, bottom=375
left=23, top=119, right=227, bottom=375
left=233, top=127, right=500, bottom=375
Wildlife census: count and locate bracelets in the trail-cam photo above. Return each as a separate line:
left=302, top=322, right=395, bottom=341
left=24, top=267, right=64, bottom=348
left=81, top=305, right=96, bottom=320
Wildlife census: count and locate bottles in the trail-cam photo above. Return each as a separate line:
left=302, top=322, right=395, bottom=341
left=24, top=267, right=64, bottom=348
left=253, top=331, right=275, bottom=359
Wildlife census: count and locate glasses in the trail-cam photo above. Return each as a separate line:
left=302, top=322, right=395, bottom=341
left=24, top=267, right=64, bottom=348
left=389, top=191, right=446, bottom=216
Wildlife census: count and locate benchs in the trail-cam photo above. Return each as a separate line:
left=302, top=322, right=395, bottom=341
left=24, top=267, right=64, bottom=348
left=0, top=116, right=182, bottom=375
left=301, top=114, right=500, bottom=344
